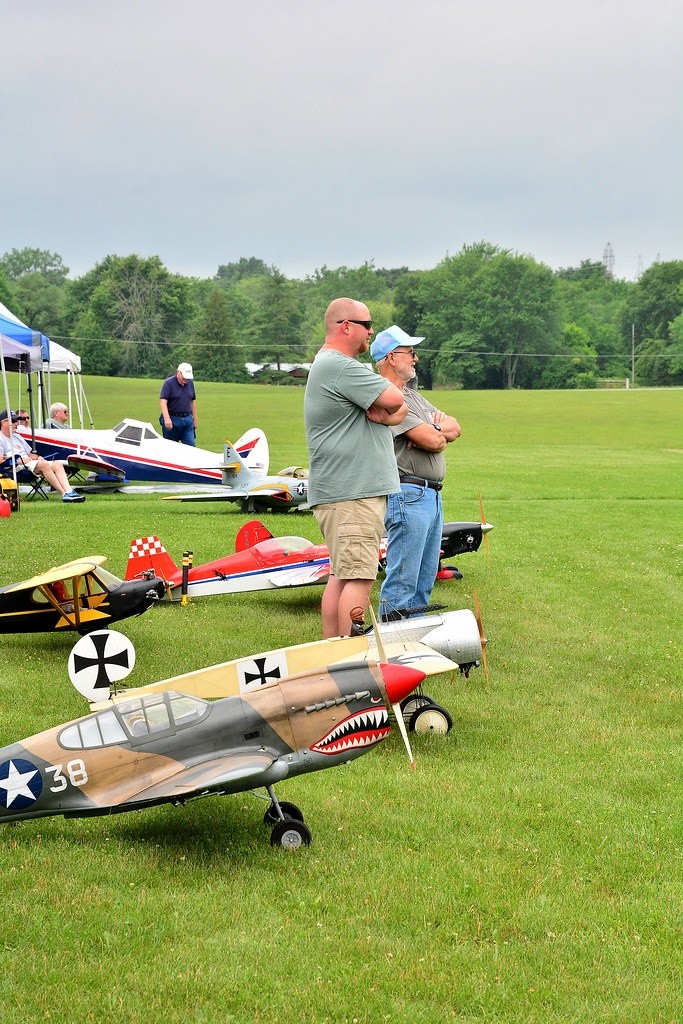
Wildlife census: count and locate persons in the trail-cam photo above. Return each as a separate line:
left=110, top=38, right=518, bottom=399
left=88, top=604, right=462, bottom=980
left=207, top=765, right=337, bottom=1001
left=159, top=362, right=198, bottom=447
left=350, top=606, right=365, bottom=636
left=0, top=402, right=86, bottom=503
left=304, top=297, right=462, bottom=641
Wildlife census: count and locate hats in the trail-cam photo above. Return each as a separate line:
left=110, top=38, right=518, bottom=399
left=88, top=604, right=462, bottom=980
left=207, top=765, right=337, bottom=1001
left=178, top=363, right=193, bottom=379
left=0, top=409, right=22, bottom=420
left=370, top=325, right=425, bottom=362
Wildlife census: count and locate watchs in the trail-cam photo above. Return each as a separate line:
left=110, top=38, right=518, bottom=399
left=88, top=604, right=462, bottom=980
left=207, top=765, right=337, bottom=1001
left=431, top=423, right=441, bottom=432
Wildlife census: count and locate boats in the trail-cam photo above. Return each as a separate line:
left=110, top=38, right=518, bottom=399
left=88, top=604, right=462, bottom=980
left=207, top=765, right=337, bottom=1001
left=13, top=419, right=224, bottom=485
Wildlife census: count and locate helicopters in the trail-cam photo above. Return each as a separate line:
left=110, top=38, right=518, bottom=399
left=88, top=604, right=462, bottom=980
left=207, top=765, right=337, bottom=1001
left=0, top=556, right=173, bottom=636
left=68, top=586, right=493, bottom=742
left=0, top=601, right=426, bottom=851
left=120, top=428, right=306, bottom=516
left=124, top=485, right=496, bottom=599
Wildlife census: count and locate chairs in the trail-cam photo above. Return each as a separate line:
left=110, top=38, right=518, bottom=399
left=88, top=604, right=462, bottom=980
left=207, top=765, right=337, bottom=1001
left=0, top=450, right=89, bottom=503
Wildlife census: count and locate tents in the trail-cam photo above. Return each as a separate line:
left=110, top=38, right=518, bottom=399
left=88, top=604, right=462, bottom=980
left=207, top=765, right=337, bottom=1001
left=0, top=302, right=95, bottom=430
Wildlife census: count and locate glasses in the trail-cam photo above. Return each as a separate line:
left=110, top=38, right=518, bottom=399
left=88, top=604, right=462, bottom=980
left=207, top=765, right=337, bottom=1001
left=337, top=320, right=372, bottom=330
left=12, top=419, right=17, bottom=423
left=385, top=351, right=415, bottom=360
left=22, top=417, right=29, bottom=420
left=64, top=411, right=66, bottom=414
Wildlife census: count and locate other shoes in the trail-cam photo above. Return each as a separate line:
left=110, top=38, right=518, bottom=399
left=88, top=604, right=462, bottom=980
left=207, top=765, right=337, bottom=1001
left=63, top=488, right=85, bottom=502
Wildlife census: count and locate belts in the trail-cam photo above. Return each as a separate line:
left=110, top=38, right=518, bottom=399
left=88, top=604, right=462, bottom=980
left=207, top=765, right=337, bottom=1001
left=400, top=477, right=442, bottom=490
